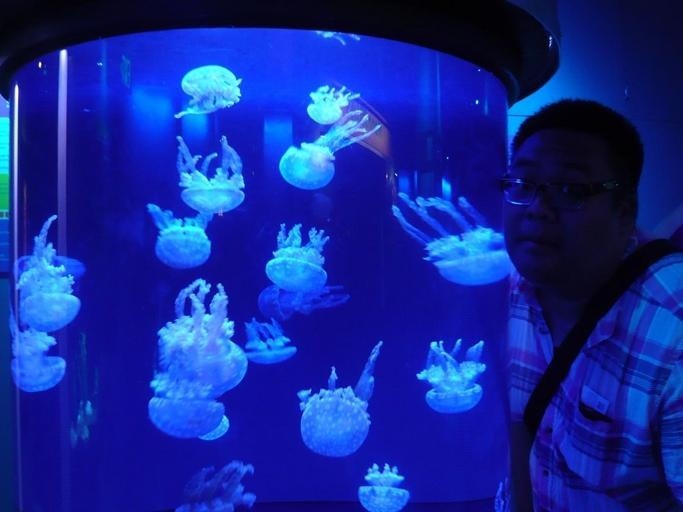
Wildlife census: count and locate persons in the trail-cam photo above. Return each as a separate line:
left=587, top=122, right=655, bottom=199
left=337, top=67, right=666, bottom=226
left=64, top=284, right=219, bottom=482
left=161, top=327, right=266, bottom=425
left=496, top=97, right=683, bottom=512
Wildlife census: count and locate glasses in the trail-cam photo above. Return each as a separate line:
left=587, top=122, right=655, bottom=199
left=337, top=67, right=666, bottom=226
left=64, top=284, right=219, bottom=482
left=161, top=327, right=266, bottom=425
left=493, top=169, right=629, bottom=210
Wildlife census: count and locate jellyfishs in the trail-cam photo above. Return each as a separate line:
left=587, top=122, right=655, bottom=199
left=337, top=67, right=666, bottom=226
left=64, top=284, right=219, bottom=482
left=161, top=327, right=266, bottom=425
left=262, top=221, right=332, bottom=292
left=416, top=339, right=488, bottom=415
left=147, top=280, right=248, bottom=444
left=174, top=63, right=244, bottom=120
left=279, top=85, right=381, bottom=191
left=173, top=462, right=257, bottom=512
left=299, top=340, right=383, bottom=456
left=146, top=135, right=248, bottom=271
left=256, top=286, right=351, bottom=325
left=357, top=462, right=411, bottom=511
left=391, top=191, right=512, bottom=286
left=244, top=315, right=300, bottom=368
left=8, top=212, right=84, bottom=394
